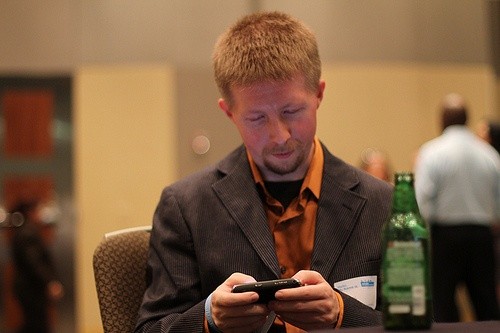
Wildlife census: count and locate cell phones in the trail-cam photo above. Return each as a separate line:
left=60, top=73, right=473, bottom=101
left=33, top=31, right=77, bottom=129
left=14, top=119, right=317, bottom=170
left=232, top=278, right=302, bottom=305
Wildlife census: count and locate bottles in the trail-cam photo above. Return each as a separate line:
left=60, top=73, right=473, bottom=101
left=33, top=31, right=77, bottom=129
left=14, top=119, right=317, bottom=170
left=377, top=172, right=435, bottom=331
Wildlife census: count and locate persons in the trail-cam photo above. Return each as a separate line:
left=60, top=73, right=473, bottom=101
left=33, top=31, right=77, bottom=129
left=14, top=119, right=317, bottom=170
left=410, top=93, right=500, bottom=322
left=361, top=147, right=393, bottom=183
left=9, top=196, right=65, bottom=333
left=129, top=10, right=395, bottom=333
